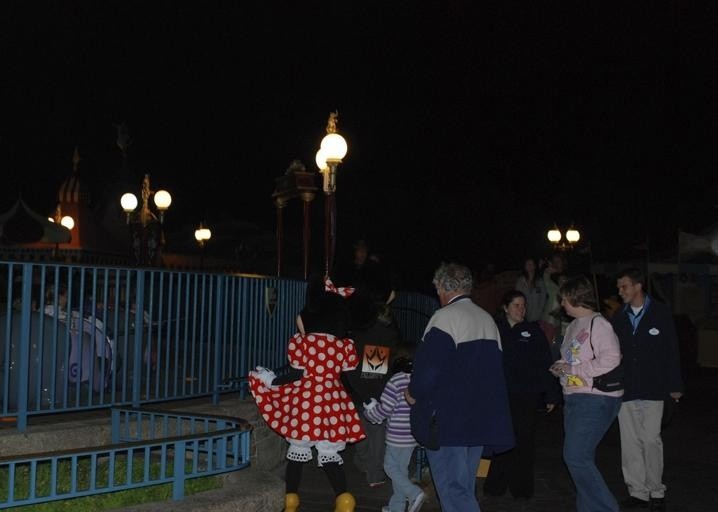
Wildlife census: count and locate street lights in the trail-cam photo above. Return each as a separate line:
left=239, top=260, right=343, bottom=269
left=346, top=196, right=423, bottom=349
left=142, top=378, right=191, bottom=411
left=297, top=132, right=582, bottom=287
left=194, top=220, right=212, bottom=266
left=548, top=222, right=581, bottom=250
left=315, top=109, right=348, bottom=276
left=48, top=216, right=75, bottom=257
left=117, top=189, right=172, bottom=270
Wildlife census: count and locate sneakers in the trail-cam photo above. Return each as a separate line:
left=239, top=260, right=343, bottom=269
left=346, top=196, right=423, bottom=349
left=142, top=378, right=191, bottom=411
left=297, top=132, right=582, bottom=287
left=407, top=491, right=426, bottom=512
left=624, top=495, right=667, bottom=512
left=482, top=467, right=536, bottom=501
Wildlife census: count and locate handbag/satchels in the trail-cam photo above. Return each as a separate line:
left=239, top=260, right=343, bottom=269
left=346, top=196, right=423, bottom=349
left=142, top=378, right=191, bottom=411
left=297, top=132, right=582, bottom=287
left=592, top=368, right=627, bottom=391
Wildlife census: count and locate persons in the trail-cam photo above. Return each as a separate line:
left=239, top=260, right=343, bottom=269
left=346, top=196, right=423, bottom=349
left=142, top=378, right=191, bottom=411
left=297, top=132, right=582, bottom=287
left=516, top=248, right=567, bottom=324
left=611, top=267, right=683, bottom=510
left=483, top=290, right=562, bottom=498
left=37, top=282, right=74, bottom=330
left=404, top=264, right=515, bottom=512
left=548, top=279, right=623, bottom=511
left=348, top=242, right=427, bottom=511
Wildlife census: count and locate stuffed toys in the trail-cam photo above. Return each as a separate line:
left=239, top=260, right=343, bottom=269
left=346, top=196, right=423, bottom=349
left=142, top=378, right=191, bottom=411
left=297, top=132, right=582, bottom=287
left=245, top=272, right=369, bottom=511
